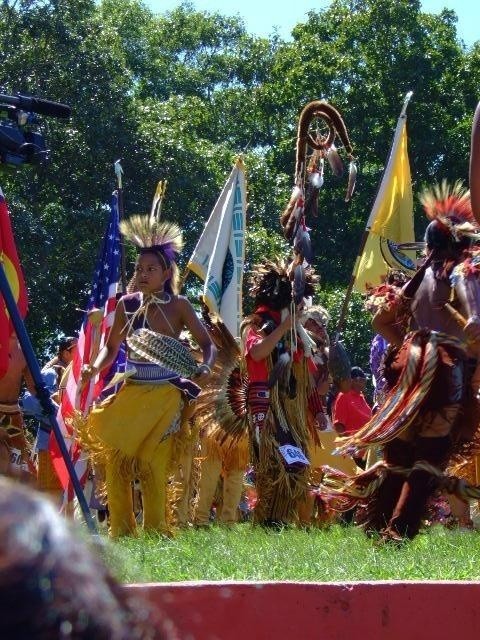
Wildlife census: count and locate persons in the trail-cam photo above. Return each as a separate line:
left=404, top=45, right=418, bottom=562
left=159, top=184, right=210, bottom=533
left=1, top=476, right=166, bottom=639
left=1, top=98, right=480, bottom=544
